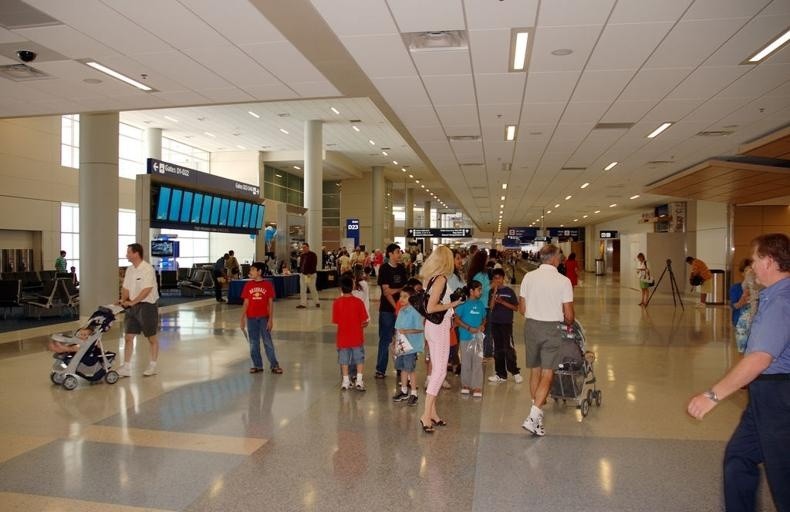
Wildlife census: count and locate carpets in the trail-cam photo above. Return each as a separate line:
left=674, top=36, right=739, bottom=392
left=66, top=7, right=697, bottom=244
left=0, top=290, right=228, bottom=333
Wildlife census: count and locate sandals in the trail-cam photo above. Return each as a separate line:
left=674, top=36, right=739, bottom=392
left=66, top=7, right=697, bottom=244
left=272, top=368, right=284, bottom=375
left=249, top=367, right=265, bottom=374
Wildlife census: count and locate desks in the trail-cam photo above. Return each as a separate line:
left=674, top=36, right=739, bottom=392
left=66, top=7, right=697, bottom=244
left=228, top=270, right=337, bottom=304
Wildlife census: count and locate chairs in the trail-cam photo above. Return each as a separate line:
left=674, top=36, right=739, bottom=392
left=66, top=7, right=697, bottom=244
left=0, top=268, right=80, bottom=320
left=155, top=262, right=215, bottom=297
left=241, top=264, right=250, bottom=279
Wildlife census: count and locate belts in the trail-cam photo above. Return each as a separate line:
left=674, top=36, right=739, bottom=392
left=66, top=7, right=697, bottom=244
left=754, top=373, right=790, bottom=382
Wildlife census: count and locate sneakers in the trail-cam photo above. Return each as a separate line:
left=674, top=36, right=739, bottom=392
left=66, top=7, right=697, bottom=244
left=216, top=298, right=226, bottom=303
left=460, top=385, right=482, bottom=398
left=113, top=365, right=133, bottom=377
left=375, top=371, right=386, bottom=379
left=316, top=303, right=321, bottom=308
left=423, top=353, right=461, bottom=390
left=481, top=356, right=494, bottom=365
left=407, top=394, right=418, bottom=407
left=694, top=302, right=706, bottom=309
left=522, top=415, right=546, bottom=436
left=513, top=374, right=525, bottom=384
left=392, top=390, right=409, bottom=402
left=637, top=301, right=648, bottom=307
left=340, top=375, right=368, bottom=393
left=142, top=363, right=160, bottom=377
left=398, top=376, right=420, bottom=389
left=295, top=304, right=307, bottom=309
left=487, top=373, right=509, bottom=384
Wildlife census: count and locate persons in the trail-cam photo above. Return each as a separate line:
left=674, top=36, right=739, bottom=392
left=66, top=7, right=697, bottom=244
left=49, top=328, right=93, bottom=354
left=687, top=233, right=790, bottom=512
left=636, top=252, right=650, bottom=306
left=685, top=256, right=713, bottom=308
left=729, top=257, right=760, bottom=390
left=212, top=243, right=581, bottom=436
left=55, top=250, right=67, bottom=273
left=70, top=267, right=79, bottom=288
left=114, top=243, right=161, bottom=377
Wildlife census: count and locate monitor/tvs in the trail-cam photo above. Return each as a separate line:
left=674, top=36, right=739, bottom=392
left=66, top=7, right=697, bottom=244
left=152, top=241, right=173, bottom=257
left=173, top=242, right=179, bottom=257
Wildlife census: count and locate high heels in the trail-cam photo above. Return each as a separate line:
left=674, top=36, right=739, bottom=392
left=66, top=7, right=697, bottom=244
left=418, top=419, right=436, bottom=434
left=432, top=418, right=448, bottom=427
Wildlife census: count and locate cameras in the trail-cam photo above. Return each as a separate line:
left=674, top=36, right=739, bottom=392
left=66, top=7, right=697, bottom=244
left=450, top=287, right=466, bottom=303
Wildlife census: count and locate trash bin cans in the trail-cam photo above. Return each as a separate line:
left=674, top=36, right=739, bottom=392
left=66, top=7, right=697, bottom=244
left=595, top=258, right=604, bottom=275
left=704, top=269, right=724, bottom=304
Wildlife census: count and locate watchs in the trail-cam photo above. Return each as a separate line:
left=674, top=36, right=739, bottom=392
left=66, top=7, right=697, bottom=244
left=707, top=387, right=721, bottom=404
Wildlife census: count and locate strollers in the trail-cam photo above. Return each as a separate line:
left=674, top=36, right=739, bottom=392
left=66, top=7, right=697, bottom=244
left=548, top=313, right=602, bottom=420
left=48, top=300, right=133, bottom=390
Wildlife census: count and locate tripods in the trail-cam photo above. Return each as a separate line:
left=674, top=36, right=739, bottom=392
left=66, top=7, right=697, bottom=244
left=645, top=259, right=685, bottom=312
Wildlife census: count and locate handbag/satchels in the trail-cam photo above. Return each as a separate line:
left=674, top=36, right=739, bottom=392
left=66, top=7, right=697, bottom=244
left=640, top=260, right=655, bottom=285
left=689, top=269, right=702, bottom=286
left=407, top=274, right=449, bottom=325
